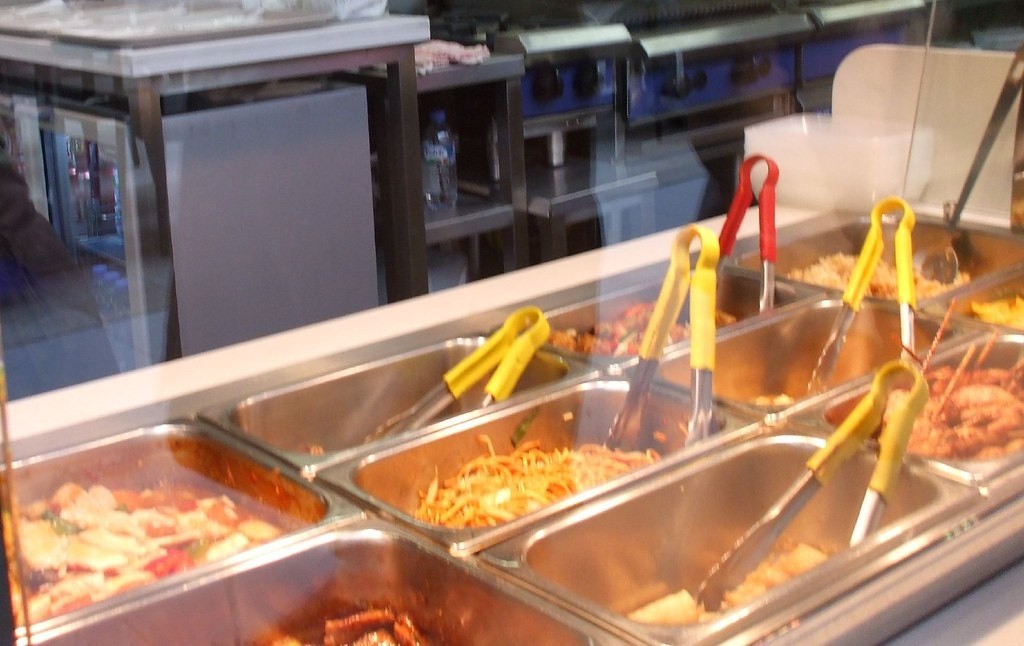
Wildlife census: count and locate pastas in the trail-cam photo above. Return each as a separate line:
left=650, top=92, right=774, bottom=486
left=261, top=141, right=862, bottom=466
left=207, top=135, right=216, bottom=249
left=413, top=434, right=658, bottom=531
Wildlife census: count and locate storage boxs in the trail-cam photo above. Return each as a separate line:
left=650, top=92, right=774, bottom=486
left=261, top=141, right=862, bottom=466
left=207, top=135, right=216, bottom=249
left=743, top=113, right=931, bottom=211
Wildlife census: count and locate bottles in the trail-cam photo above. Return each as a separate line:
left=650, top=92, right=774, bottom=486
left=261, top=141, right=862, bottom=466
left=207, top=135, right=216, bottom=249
left=422, top=110, right=458, bottom=212
left=89, top=264, right=132, bottom=363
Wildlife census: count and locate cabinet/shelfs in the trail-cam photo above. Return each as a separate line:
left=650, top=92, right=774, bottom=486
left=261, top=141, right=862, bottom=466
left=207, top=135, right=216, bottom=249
left=339, top=54, right=526, bottom=301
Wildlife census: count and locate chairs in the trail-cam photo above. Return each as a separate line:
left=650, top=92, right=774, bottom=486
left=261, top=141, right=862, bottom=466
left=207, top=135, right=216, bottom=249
left=831, top=42, right=1024, bottom=232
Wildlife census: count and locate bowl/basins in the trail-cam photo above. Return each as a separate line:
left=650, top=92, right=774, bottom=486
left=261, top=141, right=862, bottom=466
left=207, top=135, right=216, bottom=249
left=741, top=113, right=935, bottom=212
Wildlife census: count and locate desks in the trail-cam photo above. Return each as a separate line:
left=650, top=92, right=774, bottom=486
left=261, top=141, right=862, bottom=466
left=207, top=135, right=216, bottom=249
left=0, top=15, right=430, bottom=368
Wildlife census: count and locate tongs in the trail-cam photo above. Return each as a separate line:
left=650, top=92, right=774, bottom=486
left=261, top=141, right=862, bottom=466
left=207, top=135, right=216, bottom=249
left=714, top=151, right=779, bottom=322
left=363, top=304, right=550, bottom=447
left=692, top=359, right=929, bottom=613
left=806, top=195, right=916, bottom=397
left=603, top=224, right=720, bottom=453
